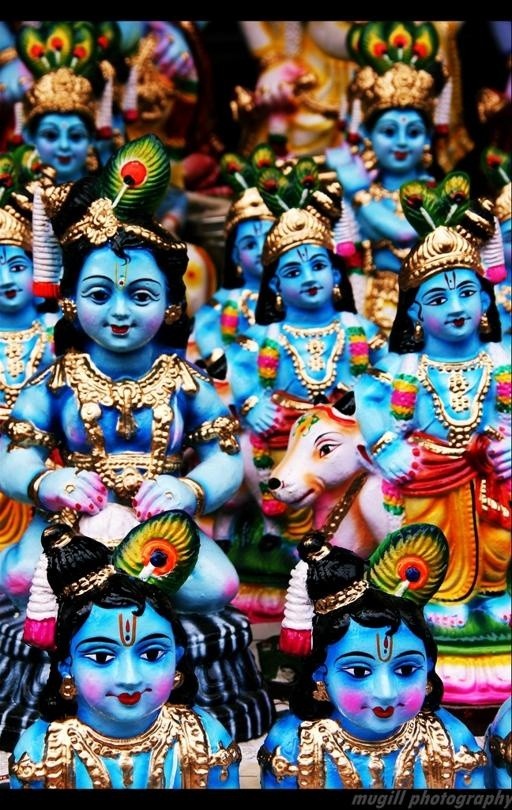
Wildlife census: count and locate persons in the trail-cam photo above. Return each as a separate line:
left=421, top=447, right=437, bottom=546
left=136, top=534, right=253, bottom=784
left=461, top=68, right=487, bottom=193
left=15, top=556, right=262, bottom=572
left=1, top=20, right=512, bottom=790
